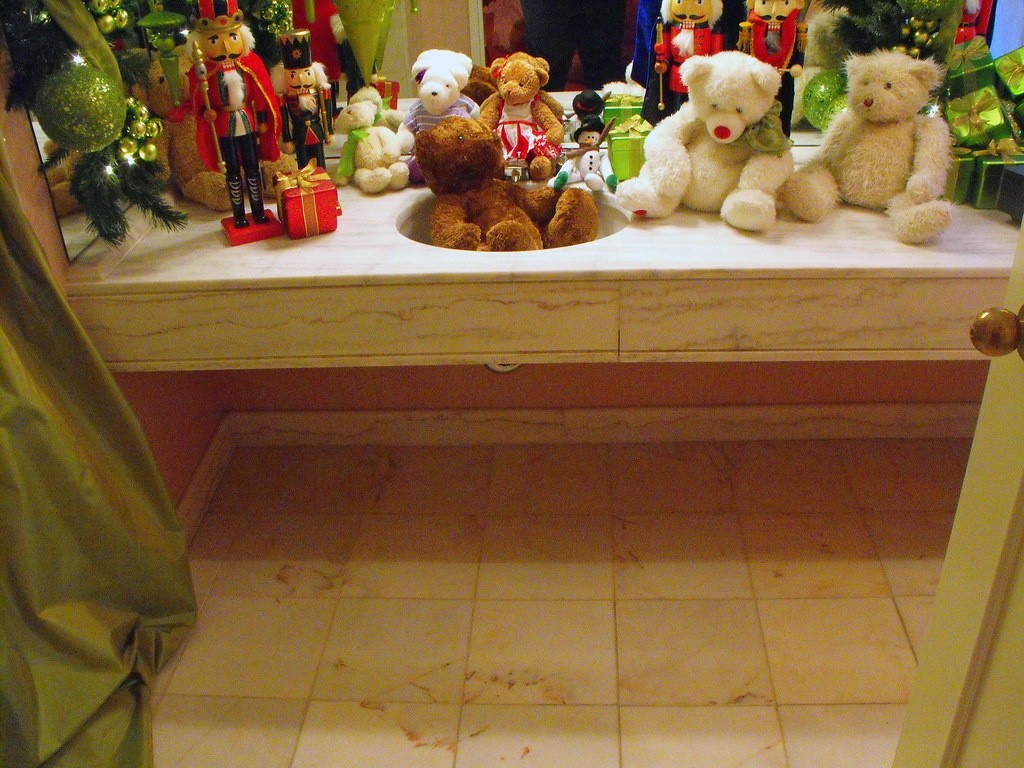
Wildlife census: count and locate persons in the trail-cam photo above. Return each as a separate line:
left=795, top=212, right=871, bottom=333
left=267, top=27, right=334, bottom=174
left=517, top=0, right=627, bottom=92
left=737, top=0, right=808, bottom=142
left=654, top=0, right=725, bottom=123
left=183, top=14, right=270, bottom=226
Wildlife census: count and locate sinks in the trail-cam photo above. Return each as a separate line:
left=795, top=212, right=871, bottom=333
left=394, top=190, right=635, bottom=254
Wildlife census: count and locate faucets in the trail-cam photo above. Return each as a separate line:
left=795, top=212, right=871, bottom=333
left=502, top=158, right=531, bottom=182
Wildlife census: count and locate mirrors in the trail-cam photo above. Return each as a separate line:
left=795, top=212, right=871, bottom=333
left=292, top=0, right=829, bottom=159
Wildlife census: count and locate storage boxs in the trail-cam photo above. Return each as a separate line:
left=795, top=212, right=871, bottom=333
left=601, top=93, right=644, bottom=131
left=915, top=33, right=1024, bottom=224
left=273, top=155, right=339, bottom=241
left=368, top=63, right=400, bottom=111
left=606, top=113, right=655, bottom=181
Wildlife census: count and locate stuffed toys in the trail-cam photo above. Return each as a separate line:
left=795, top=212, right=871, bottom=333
left=349, top=86, right=415, bottom=156
left=615, top=49, right=795, bottom=232
left=784, top=43, right=953, bottom=245
left=393, top=48, right=482, bottom=183
left=129, top=38, right=299, bottom=212
left=331, top=102, right=409, bottom=196
left=460, top=50, right=618, bottom=193
left=413, top=115, right=599, bottom=253
